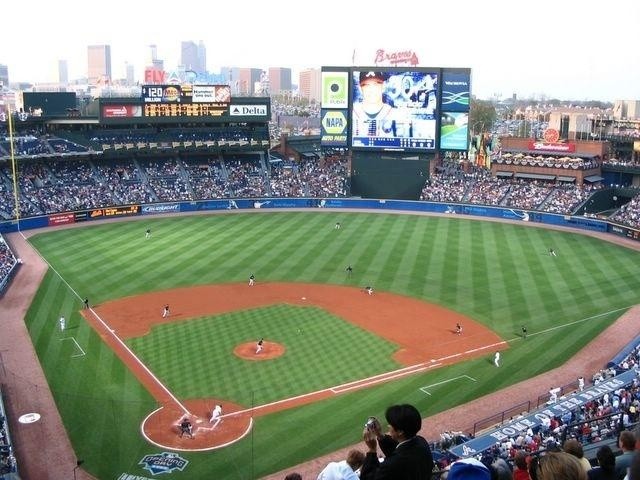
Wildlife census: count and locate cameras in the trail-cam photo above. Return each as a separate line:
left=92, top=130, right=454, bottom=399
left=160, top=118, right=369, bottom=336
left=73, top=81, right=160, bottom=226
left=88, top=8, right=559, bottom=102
left=364, top=420, right=375, bottom=431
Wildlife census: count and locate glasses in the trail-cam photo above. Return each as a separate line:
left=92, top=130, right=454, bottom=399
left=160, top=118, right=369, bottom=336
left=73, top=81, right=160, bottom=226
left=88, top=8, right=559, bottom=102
left=528, top=456, right=544, bottom=480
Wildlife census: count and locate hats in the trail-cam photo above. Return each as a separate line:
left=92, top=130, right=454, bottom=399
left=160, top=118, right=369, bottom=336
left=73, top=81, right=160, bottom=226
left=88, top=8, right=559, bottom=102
left=447, top=458, right=492, bottom=479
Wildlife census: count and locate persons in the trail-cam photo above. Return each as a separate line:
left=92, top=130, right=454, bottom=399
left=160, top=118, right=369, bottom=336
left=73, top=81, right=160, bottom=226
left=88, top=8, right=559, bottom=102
left=456, top=323, right=463, bottom=335
left=163, top=303, right=170, bottom=318
left=209, top=404, right=222, bottom=424
left=0, top=410, right=17, bottom=480
left=351, top=72, right=406, bottom=138
left=82, top=296, right=88, bottom=308
left=0, top=107, right=640, bottom=286
left=283, top=339, right=640, bottom=480
left=59, top=315, right=66, bottom=331
left=256, top=338, right=263, bottom=354
left=521, top=327, right=527, bottom=339
left=180, top=419, right=194, bottom=440
left=366, top=286, right=372, bottom=295
